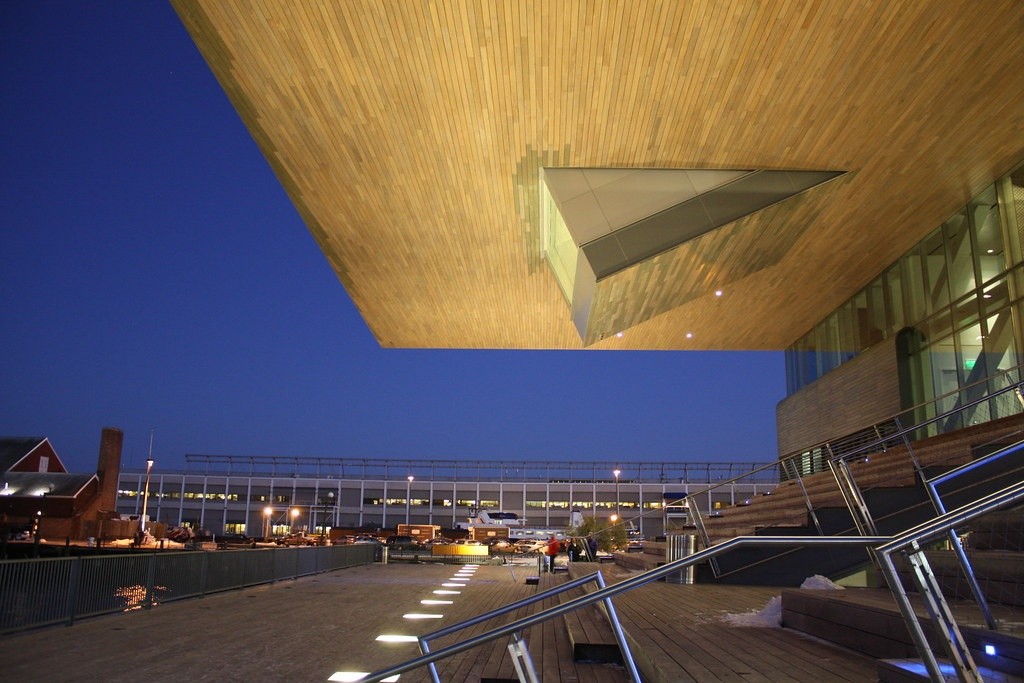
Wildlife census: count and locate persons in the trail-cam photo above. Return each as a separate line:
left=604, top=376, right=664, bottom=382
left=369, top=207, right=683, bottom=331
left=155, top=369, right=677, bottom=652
left=585, top=531, right=598, bottom=562
left=567, top=538, right=582, bottom=562
left=546, top=533, right=561, bottom=572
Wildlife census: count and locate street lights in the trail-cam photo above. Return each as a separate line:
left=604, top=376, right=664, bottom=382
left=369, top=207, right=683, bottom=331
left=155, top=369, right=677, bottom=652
left=614, top=469, right=621, bottom=517
left=142, top=459, right=154, bottom=530
left=265, top=508, right=272, bottom=541
left=408, top=476, right=414, bottom=523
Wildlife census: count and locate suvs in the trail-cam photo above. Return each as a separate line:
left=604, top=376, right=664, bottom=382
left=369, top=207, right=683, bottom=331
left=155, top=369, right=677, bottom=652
left=386, top=535, right=430, bottom=552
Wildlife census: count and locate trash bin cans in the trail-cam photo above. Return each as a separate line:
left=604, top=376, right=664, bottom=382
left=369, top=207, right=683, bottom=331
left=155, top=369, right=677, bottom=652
left=381, top=546, right=391, bottom=565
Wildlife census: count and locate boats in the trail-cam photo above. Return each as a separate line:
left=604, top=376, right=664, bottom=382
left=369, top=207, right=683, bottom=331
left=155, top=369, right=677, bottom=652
left=457, top=508, right=643, bottom=542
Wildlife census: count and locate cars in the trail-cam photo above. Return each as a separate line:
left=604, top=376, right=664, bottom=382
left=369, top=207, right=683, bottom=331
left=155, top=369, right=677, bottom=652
left=7, top=528, right=31, bottom=542
left=332, top=535, right=356, bottom=545
left=425, top=537, right=453, bottom=550
left=484, top=539, right=518, bottom=553
left=450, top=539, right=483, bottom=546
left=514, top=539, right=551, bottom=553
left=354, top=537, right=381, bottom=545
left=222, top=534, right=254, bottom=545
left=275, top=534, right=318, bottom=547
